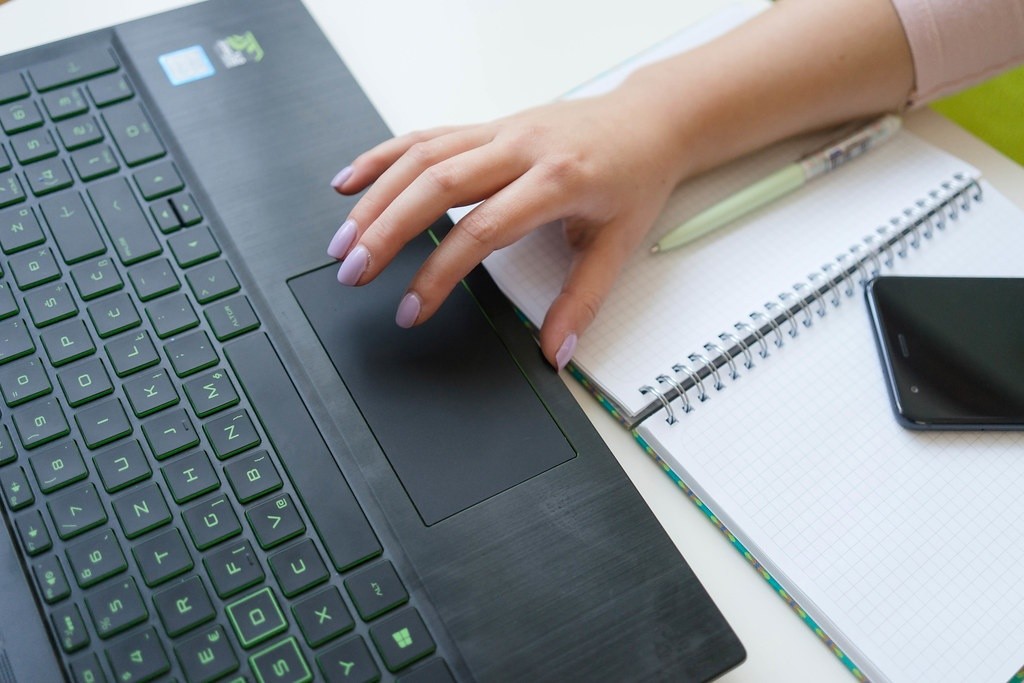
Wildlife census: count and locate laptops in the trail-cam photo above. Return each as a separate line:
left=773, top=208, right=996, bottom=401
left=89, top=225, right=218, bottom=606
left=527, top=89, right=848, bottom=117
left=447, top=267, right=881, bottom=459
left=0, top=0, right=750, bottom=683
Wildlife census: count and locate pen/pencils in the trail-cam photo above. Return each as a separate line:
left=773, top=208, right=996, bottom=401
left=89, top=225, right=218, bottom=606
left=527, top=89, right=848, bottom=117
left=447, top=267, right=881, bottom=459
left=649, top=113, right=902, bottom=255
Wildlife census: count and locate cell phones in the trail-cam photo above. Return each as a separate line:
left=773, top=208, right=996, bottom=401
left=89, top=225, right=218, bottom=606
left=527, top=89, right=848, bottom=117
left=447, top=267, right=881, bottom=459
left=866, top=276, right=1024, bottom=431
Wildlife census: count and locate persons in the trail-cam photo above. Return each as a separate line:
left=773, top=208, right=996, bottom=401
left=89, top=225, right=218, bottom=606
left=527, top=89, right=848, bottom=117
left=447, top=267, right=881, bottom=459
left=328, top=0, right=1024, bottom=375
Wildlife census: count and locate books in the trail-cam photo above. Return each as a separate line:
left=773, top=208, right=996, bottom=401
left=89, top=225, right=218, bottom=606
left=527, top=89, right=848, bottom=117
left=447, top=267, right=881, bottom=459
left=415, top=0, right=1024, bottom=683
